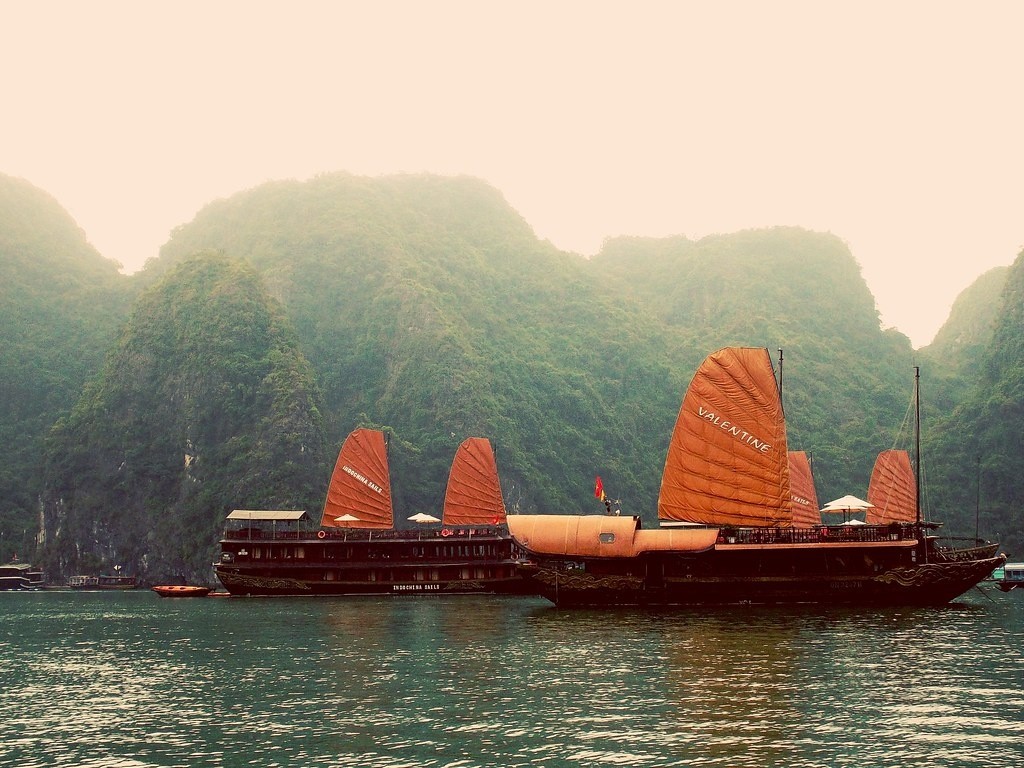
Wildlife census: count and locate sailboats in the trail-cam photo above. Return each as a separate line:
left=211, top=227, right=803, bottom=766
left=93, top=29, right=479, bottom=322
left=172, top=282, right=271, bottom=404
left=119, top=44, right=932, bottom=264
left=213, top=428, right=541, bottom=596
left=504, top=347, right=1011, bottom=612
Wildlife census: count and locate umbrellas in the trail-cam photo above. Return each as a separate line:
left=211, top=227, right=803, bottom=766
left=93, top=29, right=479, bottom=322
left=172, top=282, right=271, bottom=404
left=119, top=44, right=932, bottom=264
left=819, top=505, right=868, bottom=524
left=823, top=495, right=875, bottom=524
left=416, top=516, right=441, bottom=529
left=333, top=514, right=361, bottom=528
left=406, top=513, right=432, bottom=529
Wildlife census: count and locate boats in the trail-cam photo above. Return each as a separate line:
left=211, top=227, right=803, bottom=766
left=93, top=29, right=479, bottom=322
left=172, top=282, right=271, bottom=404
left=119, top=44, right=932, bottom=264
left=151, top=586, right=216, bottom=598
left=69, top=576, right=135, bottom=590
left=0, top=564, right=45, bottom=591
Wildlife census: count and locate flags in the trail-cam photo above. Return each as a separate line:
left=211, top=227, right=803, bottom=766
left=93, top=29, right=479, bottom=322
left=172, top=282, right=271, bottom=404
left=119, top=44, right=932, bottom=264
left=595, top=476, right=603, bottom=497
left=600, top=490, right=605, bottom=502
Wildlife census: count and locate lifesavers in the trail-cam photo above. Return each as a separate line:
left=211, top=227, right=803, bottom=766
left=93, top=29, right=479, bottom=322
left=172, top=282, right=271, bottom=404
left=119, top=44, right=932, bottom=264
left=317, top=531, right=326, bottom=539
left=511, top=553, right=518, bottom=560
left=441, top=529, right=449, bottom=537
